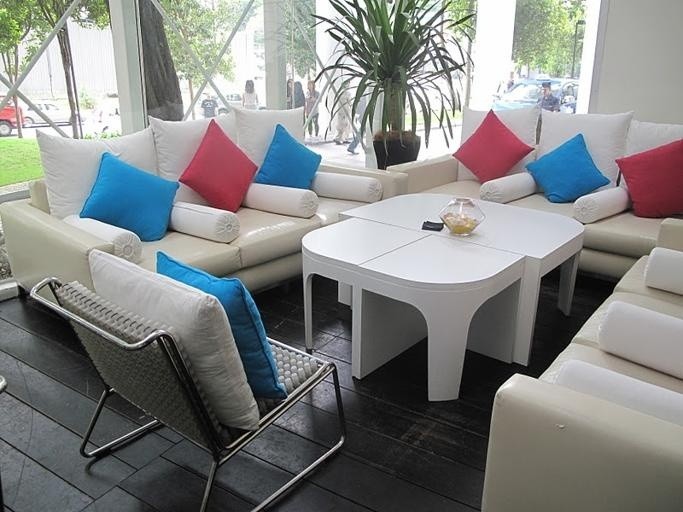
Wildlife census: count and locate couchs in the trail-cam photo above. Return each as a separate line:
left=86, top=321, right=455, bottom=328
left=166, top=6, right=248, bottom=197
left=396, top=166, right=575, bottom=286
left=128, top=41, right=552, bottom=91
left=0, top=163, right=398, bottom=296
left=480, top=214, right=682, bottom=511
left=386, top=117, right=682, bottom=287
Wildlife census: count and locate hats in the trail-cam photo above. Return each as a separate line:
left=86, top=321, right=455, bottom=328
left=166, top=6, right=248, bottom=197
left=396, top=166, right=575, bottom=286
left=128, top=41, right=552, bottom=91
left=541, top=82, right=550, bottom=87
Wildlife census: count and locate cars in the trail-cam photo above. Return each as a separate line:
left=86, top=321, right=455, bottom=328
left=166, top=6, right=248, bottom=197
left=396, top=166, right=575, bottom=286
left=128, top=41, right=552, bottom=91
left=22, top=102, right=86, bottom=128
left=492, top=78, right=579, bottom=114
left=216, top=95, right=270, bottom=115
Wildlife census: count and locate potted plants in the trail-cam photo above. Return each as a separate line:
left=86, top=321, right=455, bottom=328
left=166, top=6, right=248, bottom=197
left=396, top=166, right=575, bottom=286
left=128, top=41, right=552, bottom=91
left=315, top=0, right=476, bottom=173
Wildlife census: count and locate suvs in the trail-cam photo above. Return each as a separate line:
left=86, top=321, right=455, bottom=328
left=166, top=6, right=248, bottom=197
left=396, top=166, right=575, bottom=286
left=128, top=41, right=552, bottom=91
left=0, top=96, right=22, bottom=136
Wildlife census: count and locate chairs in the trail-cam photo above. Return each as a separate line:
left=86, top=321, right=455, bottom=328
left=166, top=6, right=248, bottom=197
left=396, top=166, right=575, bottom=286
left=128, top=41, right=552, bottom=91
left=29, top=277, right=346, bottom=510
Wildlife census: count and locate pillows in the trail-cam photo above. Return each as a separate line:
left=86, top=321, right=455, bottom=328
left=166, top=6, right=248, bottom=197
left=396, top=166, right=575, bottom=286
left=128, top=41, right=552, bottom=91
left=313, top=176, right=380, bottom=201
left=239, top=184, right=316, bottom=220
left=595, top=301, right=682, bottom=380
left=524, top=132, right=610, bottom=203
left=88, top=250, right=260, bottom=430
left=63, top=208, right=141, bottom=264
left=452, top=108, right=533, bottom=183
left=232, top=106, right=302, bottom=164
left=553, top=358, right=682, bottom=430
left=79, top=152, right=179, bottom=241
left=167, top=201, right=241, bottom=244
left=645, top=244, right=682, bottom=301
left=457, top=105, right=542, bottom=181
left=253, top=123, right=321, bottom=190
left=155, top=251, right=286, bottom=404
left=613, top=130, right=681, bottom=218
left=479, top=172, right=544, bottom=202
left=35, top=126, right=160, bottom=218
left=537, top=108, right=634, bottom=181
left=148, top=110, right=239, bottom=168
left=572, top=187, right=632, bottom=224
left=178, top=118, right=259, bottom=213
left=617, top=117, right=681, bottom=186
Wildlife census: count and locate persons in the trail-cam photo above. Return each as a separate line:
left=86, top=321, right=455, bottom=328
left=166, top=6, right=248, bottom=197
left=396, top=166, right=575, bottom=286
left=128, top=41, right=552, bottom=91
left=503, top=80, right=514, bottom=93
left=332, top=79, right=353, bottom=145
left=241, top=80, right=260, bottom=111
left=199, top=91, right=219, bottom=118
left=535, top=81, right=561, bottom=113
left=286, top=78, right=294, bottom=110
left=306, top=81, right=321, bottom=140
left=294, top=82, right=306, bottom=140
left=346, top=93, right=367, bottom=155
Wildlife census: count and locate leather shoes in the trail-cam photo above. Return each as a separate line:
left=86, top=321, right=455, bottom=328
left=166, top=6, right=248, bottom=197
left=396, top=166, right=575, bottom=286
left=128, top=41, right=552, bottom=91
left=342, top=141, right=351, bottom=144
left=346, top=148, right=359, bottom=155
left=332, top=139, right=342, bottom=145
